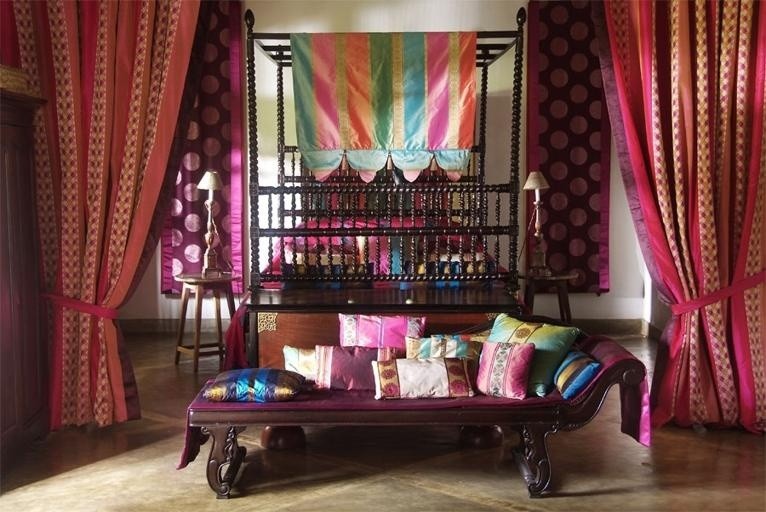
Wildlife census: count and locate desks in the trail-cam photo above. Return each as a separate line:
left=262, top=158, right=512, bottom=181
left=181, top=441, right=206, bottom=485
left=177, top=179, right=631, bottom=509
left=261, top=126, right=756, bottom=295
left=169, top=271, right=238, bottom=373
left=241, top=284, right=528, bottom=452
left=522, top=271, right=581, bottom=325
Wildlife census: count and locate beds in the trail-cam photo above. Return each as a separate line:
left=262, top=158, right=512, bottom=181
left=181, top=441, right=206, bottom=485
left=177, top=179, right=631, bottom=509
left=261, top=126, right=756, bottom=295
left=231, top=10, right=533, bottom=369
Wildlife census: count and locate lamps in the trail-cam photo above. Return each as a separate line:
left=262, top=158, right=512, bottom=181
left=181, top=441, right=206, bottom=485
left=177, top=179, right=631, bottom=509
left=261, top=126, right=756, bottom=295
left=522, top=172, right=556, bottom=276
left=194, top=170, right=227, bottom=276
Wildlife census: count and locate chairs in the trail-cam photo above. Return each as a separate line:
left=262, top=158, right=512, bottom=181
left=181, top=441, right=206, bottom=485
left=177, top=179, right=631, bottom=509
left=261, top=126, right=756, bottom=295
left=179, top=309, right=652, bottom=500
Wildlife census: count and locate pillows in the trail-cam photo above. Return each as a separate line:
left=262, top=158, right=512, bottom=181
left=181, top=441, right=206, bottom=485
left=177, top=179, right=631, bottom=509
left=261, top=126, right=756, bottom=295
left=369, top=311, right=603, bottom=403
left=336, top=311, right=425, bottom=357
left=278, top=214, right=496, bottom=289
left=308, top=341, right=397, bottom=392
left=200, top=367, right=306, bottom=403
left=283, top=341, right=318, bottom=382
